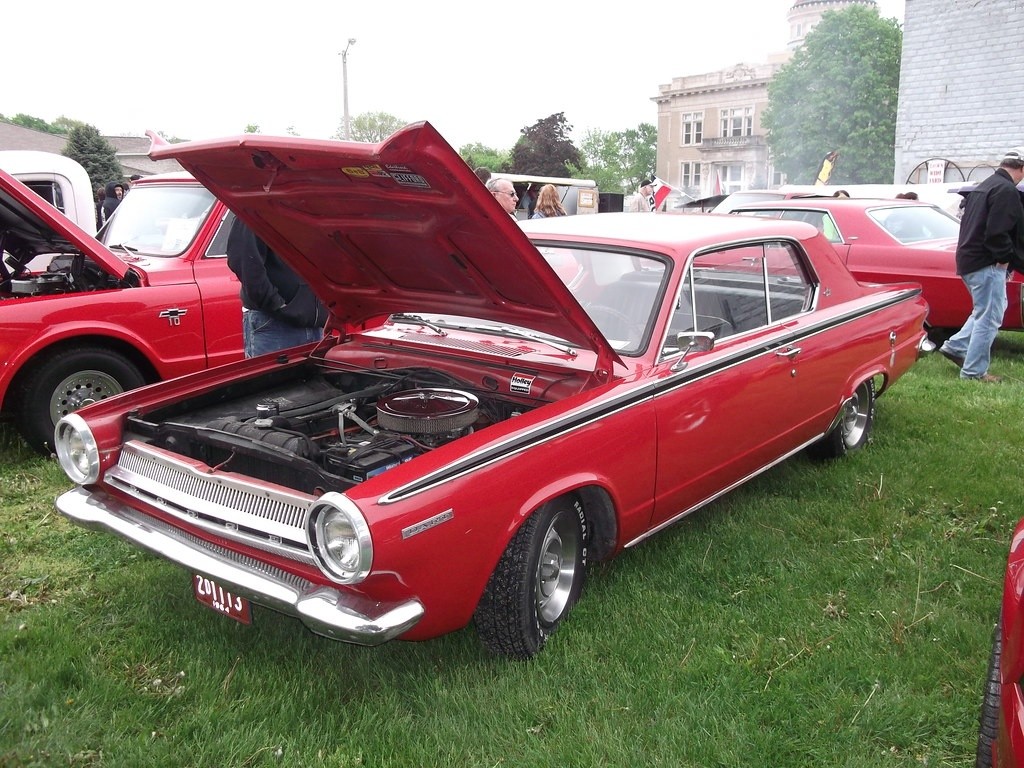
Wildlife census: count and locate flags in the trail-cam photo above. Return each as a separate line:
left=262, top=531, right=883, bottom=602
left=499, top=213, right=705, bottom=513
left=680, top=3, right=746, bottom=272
left=650, top=174, right=670, bottom=212
left=715, top=174, right=727, bottom=196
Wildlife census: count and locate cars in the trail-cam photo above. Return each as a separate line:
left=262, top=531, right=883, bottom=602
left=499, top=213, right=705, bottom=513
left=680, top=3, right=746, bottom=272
left=37, top=120, right=933, bottom=658
left=0, top=168, right=334, bottom=454
left=675, top=179, right=978, bottom=222
left=689, top=192, right=1024, bottom=336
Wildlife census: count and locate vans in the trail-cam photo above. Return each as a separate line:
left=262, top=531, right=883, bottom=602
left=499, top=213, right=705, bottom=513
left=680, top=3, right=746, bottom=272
left=487, top=173, right=601, bottom=222
left=0, top=151, right=99, bottom=247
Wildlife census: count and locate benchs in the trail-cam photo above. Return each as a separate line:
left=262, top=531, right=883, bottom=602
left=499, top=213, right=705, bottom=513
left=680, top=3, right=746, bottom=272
left=600, top=279, right=804, bottom=331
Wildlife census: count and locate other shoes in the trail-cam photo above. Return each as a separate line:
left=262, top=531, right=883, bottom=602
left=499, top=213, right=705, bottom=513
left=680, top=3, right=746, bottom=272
left=939, top=348, right=964, bottom=368
left=972, top=374, right=1001, bottom=382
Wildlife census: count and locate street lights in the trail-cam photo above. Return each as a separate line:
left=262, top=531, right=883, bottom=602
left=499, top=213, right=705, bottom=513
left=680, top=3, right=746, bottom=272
left=341, top=37, right=357, bottom=139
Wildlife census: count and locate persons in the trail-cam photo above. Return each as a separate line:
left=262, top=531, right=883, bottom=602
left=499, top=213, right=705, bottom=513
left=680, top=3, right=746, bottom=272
left=485, top=177, right=519, bottom=222
left=884, top=193, right=924, bottom=238
left=938, top=151, right=1024, bottom=382
left=475, top=167, right=491, bottom=185
left=514, top=182, right=566, bottom=219
left=629, top=180, right=657, bottom=212
left=94, top=172, right=143, bottom=232
left=227, top=215, right=328, bottom=359
left=833, top=189, right=850, bottom=197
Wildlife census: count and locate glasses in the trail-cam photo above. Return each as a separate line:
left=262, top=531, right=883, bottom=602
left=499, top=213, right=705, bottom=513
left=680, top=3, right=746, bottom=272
left=492, top=191, right=515, bottom=197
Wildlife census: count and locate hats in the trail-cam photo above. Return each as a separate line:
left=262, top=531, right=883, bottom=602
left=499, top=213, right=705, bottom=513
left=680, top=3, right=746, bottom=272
left=1003, top=146, right=1024, bottom=161
left=640, top=179, right=658, bottom=187
